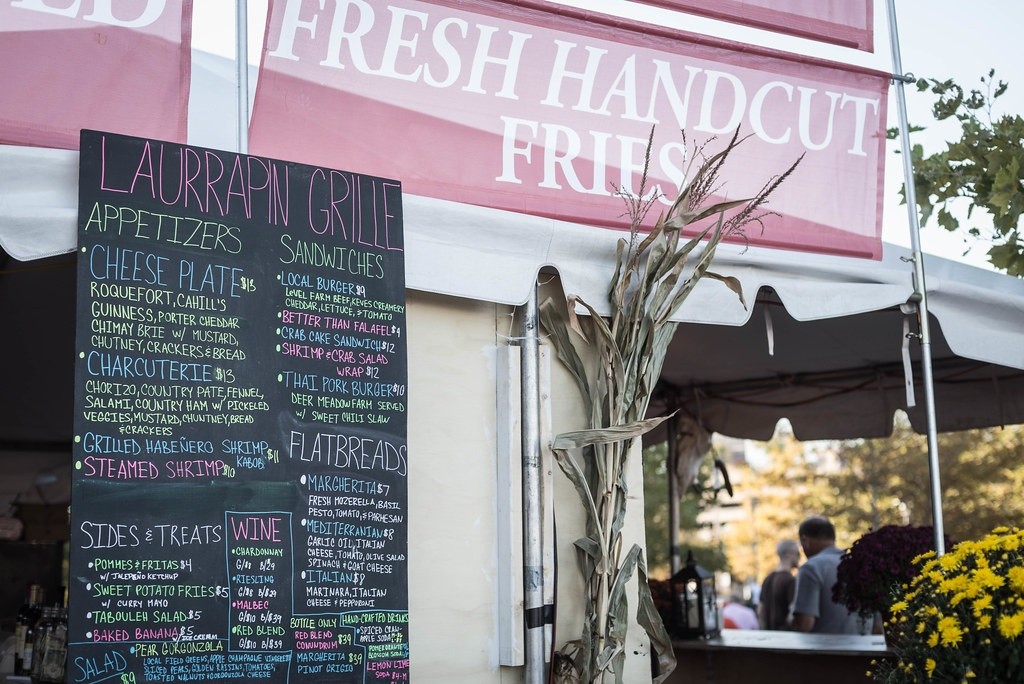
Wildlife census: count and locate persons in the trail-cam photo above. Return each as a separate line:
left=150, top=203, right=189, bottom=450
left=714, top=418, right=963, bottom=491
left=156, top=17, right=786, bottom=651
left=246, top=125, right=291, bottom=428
left=786, top=517, right=884, bottom=636
left=708, top=539, right=801, bottom=631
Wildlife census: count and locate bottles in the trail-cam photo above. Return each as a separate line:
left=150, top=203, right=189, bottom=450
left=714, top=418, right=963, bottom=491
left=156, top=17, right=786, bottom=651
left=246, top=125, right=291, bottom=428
left=14, top=585, right=66, bottom=674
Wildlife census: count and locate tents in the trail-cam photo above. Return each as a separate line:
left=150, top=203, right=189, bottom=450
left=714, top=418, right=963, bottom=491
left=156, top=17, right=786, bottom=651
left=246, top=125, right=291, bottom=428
left=0, top=24, right=1024, bottom=684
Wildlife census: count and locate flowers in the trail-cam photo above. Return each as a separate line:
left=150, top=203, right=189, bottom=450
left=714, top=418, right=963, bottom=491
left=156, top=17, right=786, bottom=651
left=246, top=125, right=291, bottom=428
left=831, top=523, right=958, bottom=633
left=867, top=525, right=1024, bottom=684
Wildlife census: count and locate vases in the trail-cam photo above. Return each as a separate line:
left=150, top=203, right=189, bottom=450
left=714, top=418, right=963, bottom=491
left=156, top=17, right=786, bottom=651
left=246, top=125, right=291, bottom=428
left=880, top=611, right=897, bottom=648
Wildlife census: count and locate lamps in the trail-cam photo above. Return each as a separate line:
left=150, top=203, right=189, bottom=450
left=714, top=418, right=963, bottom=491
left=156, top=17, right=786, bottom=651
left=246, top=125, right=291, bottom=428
left=667, top=549, right=720, bottom=643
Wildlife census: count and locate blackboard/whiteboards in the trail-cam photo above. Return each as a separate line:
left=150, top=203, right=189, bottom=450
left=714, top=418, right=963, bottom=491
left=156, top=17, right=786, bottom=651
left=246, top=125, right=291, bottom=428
left=61, top=130, right=410, bottom=684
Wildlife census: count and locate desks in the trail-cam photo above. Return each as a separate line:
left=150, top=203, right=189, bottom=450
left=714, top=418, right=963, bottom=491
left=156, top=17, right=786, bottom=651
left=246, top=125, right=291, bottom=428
left=659, top=628, right=897, bottom=684
